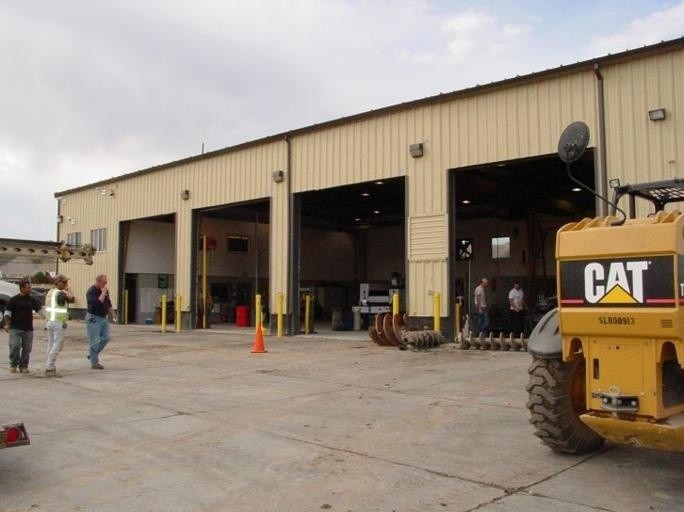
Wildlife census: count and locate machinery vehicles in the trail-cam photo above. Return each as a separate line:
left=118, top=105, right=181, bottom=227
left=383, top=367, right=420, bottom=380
left=525, top=117, right=684, bottom=460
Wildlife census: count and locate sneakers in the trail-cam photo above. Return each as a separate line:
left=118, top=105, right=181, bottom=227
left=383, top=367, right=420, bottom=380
left=46, top=370, right=63, bottom=378
left=92, top=363, right=103, bottom=369
left=20, top=369, right=29, bottom=373
left=10, top=367, right=16, bottom=373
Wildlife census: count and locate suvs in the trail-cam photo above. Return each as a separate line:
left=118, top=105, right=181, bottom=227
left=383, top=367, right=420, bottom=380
left=0, top=292, right=14, bottom=328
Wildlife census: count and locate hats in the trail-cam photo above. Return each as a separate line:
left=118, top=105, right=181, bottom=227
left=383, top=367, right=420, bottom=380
left=55, top=275, right=70, bottom=284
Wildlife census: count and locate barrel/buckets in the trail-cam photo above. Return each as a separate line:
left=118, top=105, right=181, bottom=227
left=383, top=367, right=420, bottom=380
left=236, top=305, right=249, bottom=327
left=211, top=295, right=221, bottom=324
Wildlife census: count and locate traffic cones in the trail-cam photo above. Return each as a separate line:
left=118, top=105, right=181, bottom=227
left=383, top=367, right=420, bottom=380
left=248, top=324, right=269, bottom=355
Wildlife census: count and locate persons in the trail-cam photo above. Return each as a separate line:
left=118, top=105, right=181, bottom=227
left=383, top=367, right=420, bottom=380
left=507, top=281, right=528, bottom=338
left=43, top=275, right=75, bottom=379
left=3, top=279, right=49, bottom=374
left=473, top=277, right=490, bottom=339
left=483, top=278, right=501, bottom=336
left=83, top=274, right=117, bottom=370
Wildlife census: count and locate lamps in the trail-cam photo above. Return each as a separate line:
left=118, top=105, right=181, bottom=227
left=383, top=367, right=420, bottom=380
left=410, top=143, right=423, bottom=157
left=180, top=190, right=189, bottom=200
left=273, top=171, right=283, bottom=182
left=609, top=179, right=620, bottom=188
left=56, top=215, right=63, bottom=223
left=648, top=108, right=665, bottom=121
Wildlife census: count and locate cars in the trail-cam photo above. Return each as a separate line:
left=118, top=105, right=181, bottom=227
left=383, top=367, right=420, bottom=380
left=29, top=289, right=47, bottom=308
